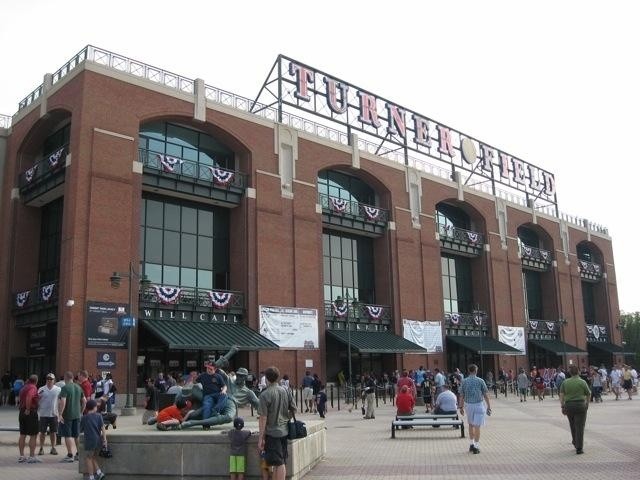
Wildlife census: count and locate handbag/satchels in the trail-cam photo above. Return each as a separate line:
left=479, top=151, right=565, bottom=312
left=99, top=446, right=113, bottom=458
left=288, top=421, right=307, bottom=440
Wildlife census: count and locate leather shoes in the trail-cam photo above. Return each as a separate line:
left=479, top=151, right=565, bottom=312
left=469, top=445, right=474, bottom=452
left=473, top=448, right=481, bottom=454
left=577, top=449, right=584, bottom=454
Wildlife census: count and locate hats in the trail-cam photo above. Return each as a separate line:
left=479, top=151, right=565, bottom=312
left=260, top=367, right=280, bottom=378
left=46, top=373, right=56, bottom=379
left=145, top=378, right=154, bottom=382
left=234, top=418, right=244, bottom=426
left=204, top=362, right=217, bottom=369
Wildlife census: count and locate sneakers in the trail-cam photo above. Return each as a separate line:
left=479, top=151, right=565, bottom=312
left=18, top=449, right=79, bottom=464
left=95, top=472, right=104, bottom=480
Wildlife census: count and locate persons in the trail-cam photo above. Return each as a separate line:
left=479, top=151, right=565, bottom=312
left=2, top=369, right=120, bottom=480
left=559, top=365, right=591, bottom=454
left=579, top=362, right=639, bottom=402
left=302, top=370, right=377, bottom=420
left=379, top=362, right=466, bottom=428
left=141, top=361, right=299, bottom=480
left=498, top=365, right=571, bottom=402
left=331, top=296, right=344, bottom=321
left=458, top=364, right=492, bottom=454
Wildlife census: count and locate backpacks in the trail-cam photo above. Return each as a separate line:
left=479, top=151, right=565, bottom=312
left=14, top=381, right=22, bottom=393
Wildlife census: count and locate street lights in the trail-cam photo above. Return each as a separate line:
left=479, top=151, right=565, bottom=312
left=554, top=316, right=570, bottom=375
left=334, top=288, right=359, bottom=398
left=110, top=262, right=151, bottom=409
left=470, top=303, right=487, bottom=379
left=616, top=322, right=628, bottom=364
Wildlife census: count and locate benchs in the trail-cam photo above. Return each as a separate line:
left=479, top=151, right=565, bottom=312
left=392, top=420, right=464, bottom=438
left=396, top=414, right=459, bottom=430
